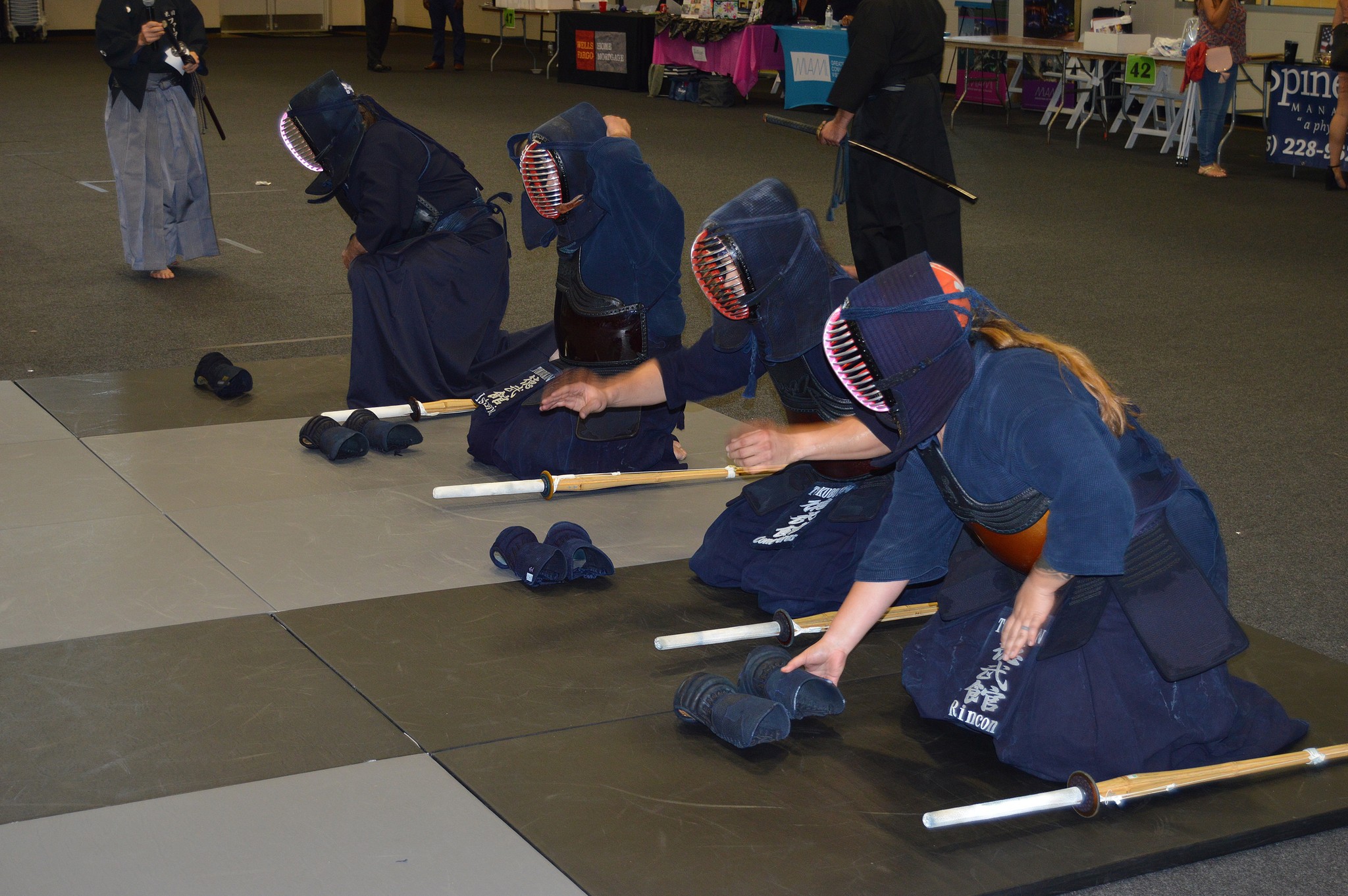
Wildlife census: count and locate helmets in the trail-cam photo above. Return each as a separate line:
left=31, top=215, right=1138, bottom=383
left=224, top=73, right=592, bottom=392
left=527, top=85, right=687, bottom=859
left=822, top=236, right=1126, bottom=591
left=519, top=138, right=564, bottom=221
left=278, top=82, right=354, bottom=172
left=689, top=225, right=751, bottom=321
left=820, top=260, right=972, bottom=413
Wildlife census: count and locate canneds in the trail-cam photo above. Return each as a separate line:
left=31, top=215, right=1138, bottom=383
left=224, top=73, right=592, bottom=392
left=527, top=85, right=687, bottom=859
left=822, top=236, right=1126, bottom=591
left=660, top=4, right=667, bottom=14
left=481, top=38, right=490, bottom=43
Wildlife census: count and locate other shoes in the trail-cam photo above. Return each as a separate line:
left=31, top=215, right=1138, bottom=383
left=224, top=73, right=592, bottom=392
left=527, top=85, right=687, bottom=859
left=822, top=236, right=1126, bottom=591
left=454, top=62, right=464, bottom=70
left=369, top=63, right=391, bottom=72
left=424, top=61, right=444, bottom=70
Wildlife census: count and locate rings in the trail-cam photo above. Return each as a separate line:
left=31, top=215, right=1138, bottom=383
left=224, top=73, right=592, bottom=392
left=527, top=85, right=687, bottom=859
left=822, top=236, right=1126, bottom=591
left=1020, top=626, right=1029, bottom=631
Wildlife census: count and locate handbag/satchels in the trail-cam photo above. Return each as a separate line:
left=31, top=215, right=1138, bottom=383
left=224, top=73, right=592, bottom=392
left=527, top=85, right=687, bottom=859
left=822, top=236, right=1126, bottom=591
left=1204, top=45, right=1232, bottom=73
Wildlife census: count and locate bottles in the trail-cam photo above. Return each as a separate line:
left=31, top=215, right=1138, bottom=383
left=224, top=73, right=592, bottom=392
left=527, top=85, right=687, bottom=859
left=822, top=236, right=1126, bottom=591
left=825, top=5, right=833, bottom=28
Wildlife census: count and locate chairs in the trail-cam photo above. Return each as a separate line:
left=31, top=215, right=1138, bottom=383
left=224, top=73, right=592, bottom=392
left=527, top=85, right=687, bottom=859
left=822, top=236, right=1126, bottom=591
left=1124, top=63, right=1201, bottom=153
left=1039, top=55, right=1107, bottom=128
left=1109, top=66, right=1177, bottom=134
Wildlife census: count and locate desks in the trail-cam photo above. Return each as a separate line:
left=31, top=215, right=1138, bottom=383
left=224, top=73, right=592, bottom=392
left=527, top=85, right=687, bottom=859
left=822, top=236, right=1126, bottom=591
left=479, top=5, right=1348, bottom=177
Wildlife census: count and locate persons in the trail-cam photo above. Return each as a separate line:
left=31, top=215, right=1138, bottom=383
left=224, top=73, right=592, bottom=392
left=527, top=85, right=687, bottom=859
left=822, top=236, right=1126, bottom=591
left=281, top=70, right=553, bottom=409
left=364, top=0, right=393, bottom=72
left=780, top=251, right=1308, bottom=784
left=761, top=0, right=964, bottom=289
left=1194, top=0, right=1247, bottom=178
left=94, top=0, right=220, bottom=279
left=466, top=99, right=689, bottom=480
left=1329, top=0, right=1348, bottom=190
left=422, top=0, right=466, bottom=71
left=539, top=178, right=943, bottom=621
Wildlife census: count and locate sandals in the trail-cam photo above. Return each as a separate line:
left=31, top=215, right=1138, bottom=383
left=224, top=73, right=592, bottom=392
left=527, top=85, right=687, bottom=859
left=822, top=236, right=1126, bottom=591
left=1198, top=162, right=1227, bottom=177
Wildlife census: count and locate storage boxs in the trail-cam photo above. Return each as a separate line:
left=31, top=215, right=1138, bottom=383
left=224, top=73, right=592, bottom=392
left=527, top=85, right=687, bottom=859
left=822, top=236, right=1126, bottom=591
left=1084, top=31, right=1152, bottom=56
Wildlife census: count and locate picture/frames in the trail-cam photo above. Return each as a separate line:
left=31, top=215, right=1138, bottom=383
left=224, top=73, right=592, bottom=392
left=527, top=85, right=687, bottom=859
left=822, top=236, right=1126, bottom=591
left=1312, top=21, right=1334, bottom=63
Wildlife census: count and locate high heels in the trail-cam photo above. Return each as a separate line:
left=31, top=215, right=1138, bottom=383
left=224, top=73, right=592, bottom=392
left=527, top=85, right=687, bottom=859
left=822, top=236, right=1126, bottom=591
left=1326, top=164, right=1348, bottom=190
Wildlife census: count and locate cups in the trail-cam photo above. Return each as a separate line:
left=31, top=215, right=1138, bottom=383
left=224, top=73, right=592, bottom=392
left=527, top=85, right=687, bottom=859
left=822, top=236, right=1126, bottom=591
left=1284, top=40, right=1299, bottom=66
left=599, top=1, right=607, bottom=12
